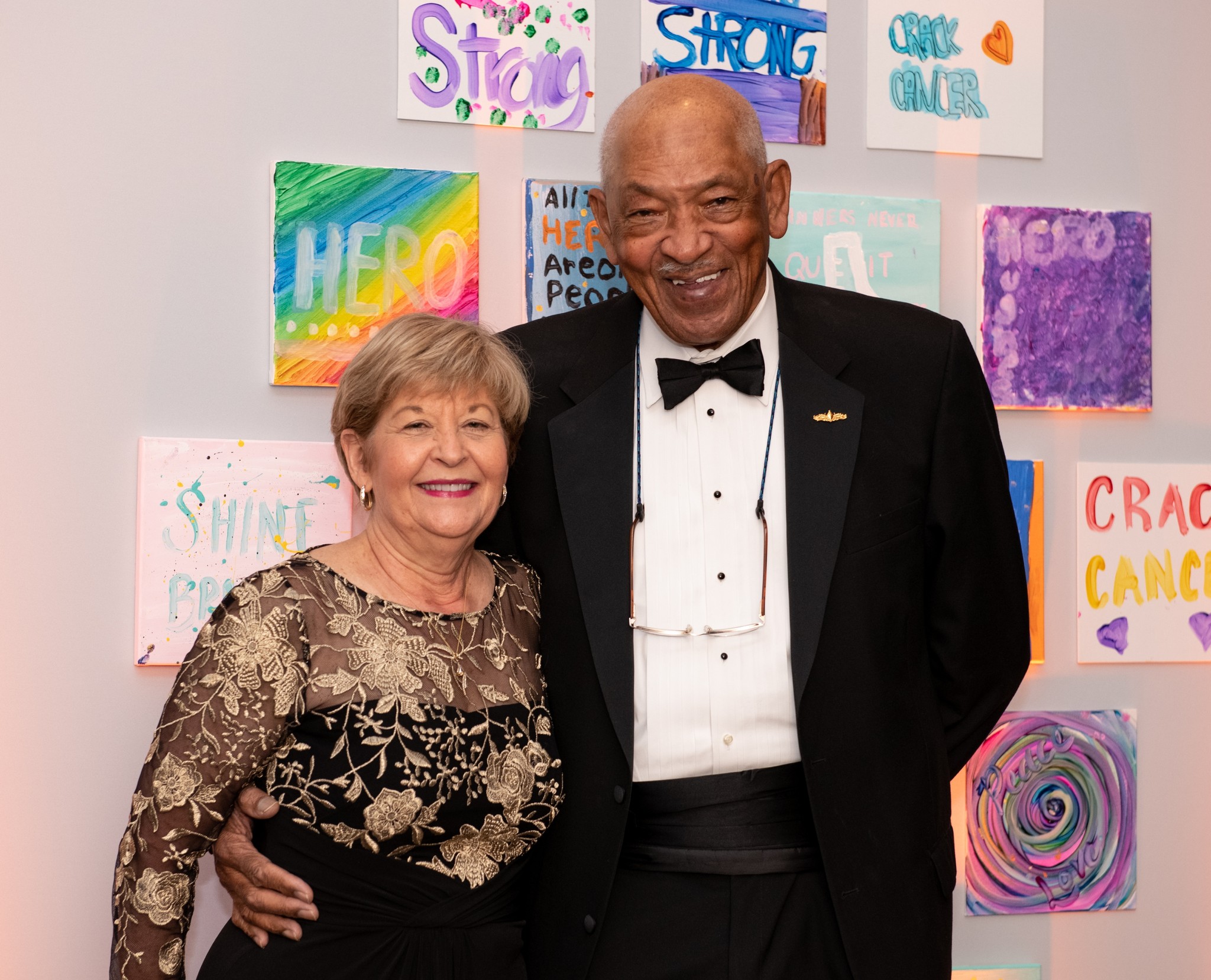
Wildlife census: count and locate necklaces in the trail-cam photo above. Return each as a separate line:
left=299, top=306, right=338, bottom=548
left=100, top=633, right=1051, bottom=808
left=362, top=529, right=470, bottom=677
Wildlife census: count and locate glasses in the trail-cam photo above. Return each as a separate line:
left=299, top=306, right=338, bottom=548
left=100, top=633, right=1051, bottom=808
left=628, top=499, right=768, bottom=637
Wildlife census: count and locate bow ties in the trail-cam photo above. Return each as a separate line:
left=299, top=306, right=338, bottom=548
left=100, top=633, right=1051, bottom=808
left=654, top=338, right=766, bottom=410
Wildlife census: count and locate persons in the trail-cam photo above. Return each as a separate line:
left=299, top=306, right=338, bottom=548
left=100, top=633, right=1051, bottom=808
left=103, top=319, right=564, bottom=976
left=209, top=71, right=1031, bottom=979
